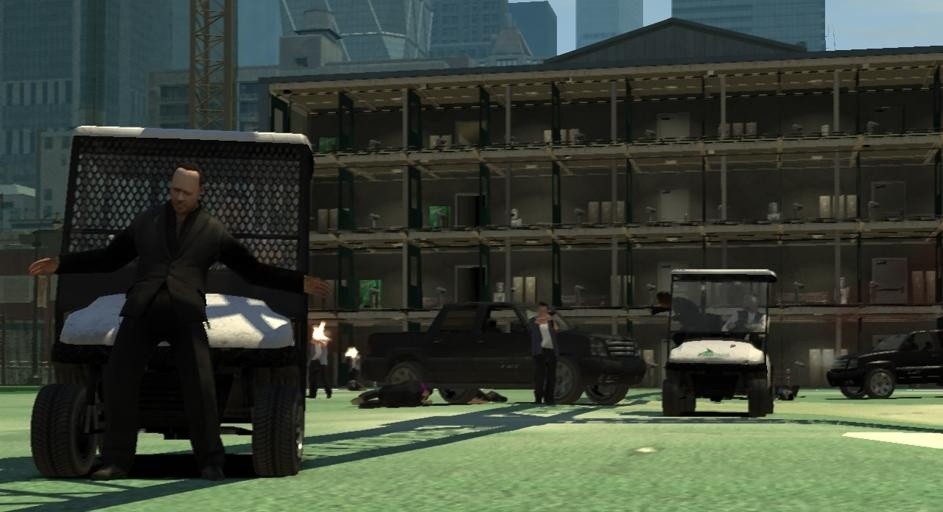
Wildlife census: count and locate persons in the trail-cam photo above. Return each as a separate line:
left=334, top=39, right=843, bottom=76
left=351, top=380, right=432, bottom=408
left=650, top=290, right=701, bottom=343
left=724, top=293, right=770, bottom=346
left=29, top=160, right=332, bottom=481
left=306, top=322, right=333, bottom=399
left=466, top=388, right=509, bottom=405
left=340, top=342, right=362, bottom=385
left=528, top=300, right=561, bottom=407
